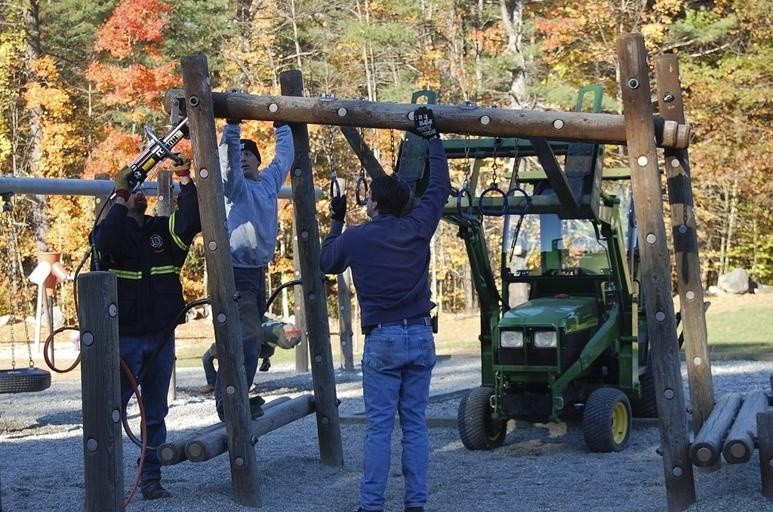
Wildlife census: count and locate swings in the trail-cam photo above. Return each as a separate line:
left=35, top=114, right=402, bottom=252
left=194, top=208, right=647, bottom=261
left=0, top=194, right=50, bottom=392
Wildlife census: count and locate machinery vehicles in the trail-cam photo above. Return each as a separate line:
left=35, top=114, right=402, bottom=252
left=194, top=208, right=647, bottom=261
left=339, top=80, right=711, bottom=456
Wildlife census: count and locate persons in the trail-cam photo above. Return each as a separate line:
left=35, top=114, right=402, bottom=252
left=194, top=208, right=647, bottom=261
left=318, top=104, right=452, bottom=512
left=201, top=350, right=218, bottom=395
left=87, top=152, right=199, bottom=500
left=211, top=87, right=297, bottom=425
left=256, top=313, right=276, bottom=371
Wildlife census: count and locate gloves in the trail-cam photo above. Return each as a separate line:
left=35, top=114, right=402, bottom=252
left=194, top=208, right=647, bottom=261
left=409, top=107, right=439, bottom=142
left=170, top=154, right=191, bottom=177
left=115, top=166, right=133, bottom=202
left=329, top=195, right=346, bottom=223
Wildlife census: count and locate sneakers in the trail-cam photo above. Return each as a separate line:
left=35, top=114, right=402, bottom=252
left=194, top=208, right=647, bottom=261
left=141, top=480, right=171, bottom=500
left=260, top=360, right=270, bottom=371
left=219, top=396, right=265, bottom=422
left=200, top=384, right=215, bottom=393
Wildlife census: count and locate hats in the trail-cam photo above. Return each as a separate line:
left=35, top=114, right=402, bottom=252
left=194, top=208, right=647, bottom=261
left=240, top=139, right=261, bottom=167
